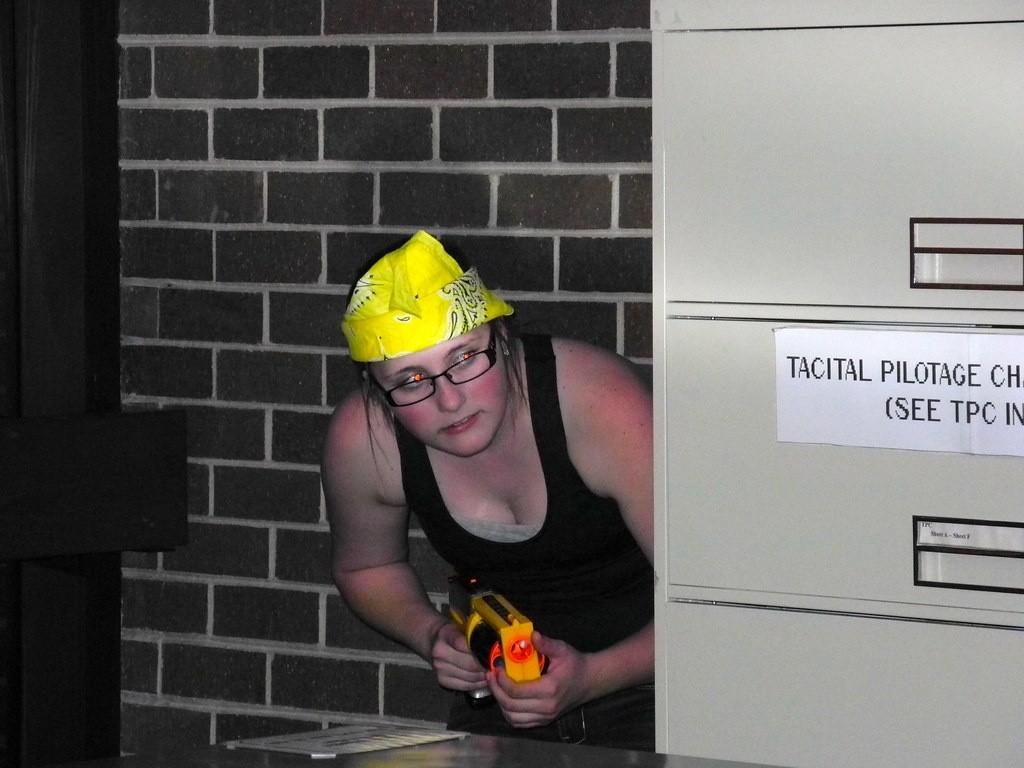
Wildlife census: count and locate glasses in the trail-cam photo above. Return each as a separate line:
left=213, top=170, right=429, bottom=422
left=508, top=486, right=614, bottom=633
left=368, top=321, right=496, bottom=406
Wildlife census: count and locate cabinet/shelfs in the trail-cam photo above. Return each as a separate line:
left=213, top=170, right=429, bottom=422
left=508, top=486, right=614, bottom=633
left=650, top=0, right=1023, bottom=768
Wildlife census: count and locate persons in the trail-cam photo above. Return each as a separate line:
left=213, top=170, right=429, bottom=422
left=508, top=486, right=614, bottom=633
left=319, top=228, right=656, bottom=752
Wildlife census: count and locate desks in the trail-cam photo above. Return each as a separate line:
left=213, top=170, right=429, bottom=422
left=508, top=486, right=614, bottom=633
left=103, top=723, right=770, bottom=768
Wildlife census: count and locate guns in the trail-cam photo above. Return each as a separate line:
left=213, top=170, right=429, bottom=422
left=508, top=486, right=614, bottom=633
left=444, top=562, right=547, bottom=713
